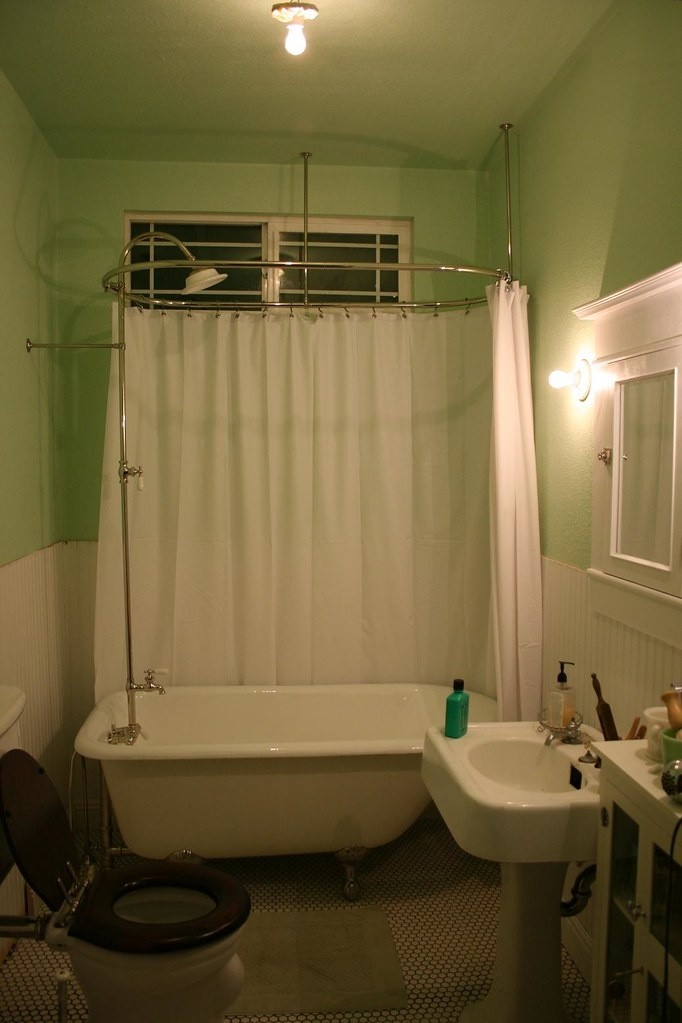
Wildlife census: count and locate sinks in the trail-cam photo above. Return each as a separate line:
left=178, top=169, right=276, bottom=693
left=421, top=718, right=618, bottom=862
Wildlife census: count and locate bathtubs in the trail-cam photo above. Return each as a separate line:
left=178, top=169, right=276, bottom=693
left=73, top=682, right=499, bottom=860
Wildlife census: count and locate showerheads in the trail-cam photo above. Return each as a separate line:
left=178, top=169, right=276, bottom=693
left=179, top=268, right=227, bottom=295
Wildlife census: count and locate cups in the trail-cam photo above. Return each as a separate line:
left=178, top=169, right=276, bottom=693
left=643, top=706, right=670, bottom=761
left=661, top=728, right=682, bottom=766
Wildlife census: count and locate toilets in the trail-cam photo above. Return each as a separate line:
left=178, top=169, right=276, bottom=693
left=0, top=747, right=254, bottom=1023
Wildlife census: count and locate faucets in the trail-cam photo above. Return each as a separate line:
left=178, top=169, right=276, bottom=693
left=131, top=668, right=165, bottom=695
left=543, top=717, right=585, bottom=749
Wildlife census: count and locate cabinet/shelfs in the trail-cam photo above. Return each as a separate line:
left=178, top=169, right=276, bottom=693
left=583, top=740, right=682, bottom=1023
left=570, top=262, right=681, bottom=598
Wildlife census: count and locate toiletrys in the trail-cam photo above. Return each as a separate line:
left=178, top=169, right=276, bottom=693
left=445, top=679, right=469, bottom=737
left=545, top=661, right=573, bottom=731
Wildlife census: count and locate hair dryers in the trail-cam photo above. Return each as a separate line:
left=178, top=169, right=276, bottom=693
left=659, top=756, right=681, bottom=809
